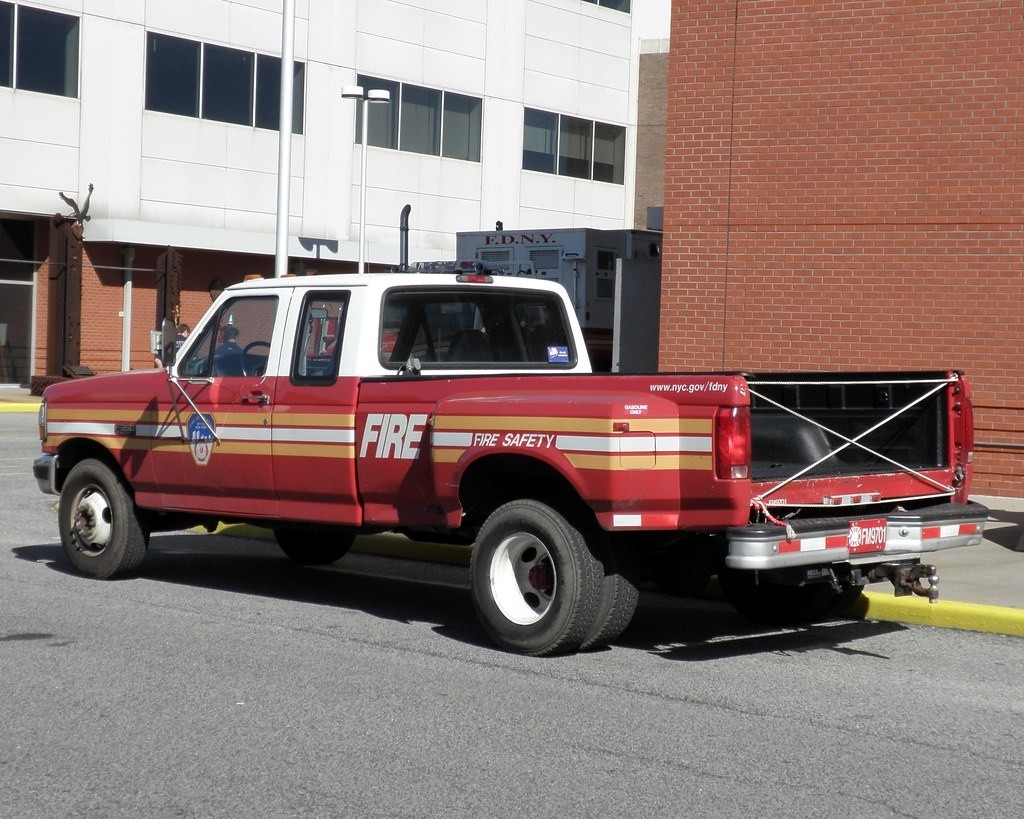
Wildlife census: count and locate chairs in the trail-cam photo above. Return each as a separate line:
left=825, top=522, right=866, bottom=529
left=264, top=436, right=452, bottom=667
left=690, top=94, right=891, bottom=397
left=443, top=329, right=496, bottom=362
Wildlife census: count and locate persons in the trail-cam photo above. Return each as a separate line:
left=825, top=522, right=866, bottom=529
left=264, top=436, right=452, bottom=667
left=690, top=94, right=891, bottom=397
left=154, top=323, right=199, bottom=369
left=213, top=326, right=245, bottom=376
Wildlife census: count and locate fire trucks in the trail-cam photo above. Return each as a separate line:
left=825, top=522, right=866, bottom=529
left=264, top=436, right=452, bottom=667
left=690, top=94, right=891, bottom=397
left=307, top=203, right=663, bottom=374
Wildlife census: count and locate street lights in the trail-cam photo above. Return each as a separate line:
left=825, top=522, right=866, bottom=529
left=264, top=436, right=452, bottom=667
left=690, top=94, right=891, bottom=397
left=339, top=84, right=392, bottom=274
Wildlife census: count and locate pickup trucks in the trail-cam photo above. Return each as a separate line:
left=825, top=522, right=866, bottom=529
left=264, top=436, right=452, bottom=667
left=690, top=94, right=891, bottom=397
left=29, top=267, right=993, bottom=660
